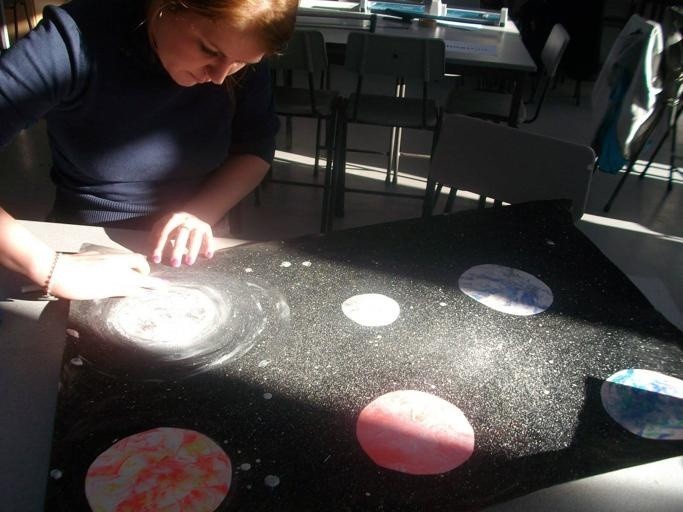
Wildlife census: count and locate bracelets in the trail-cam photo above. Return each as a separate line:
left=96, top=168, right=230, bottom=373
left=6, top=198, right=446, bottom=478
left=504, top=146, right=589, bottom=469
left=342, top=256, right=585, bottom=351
left=41, top=251, right=60, bottom=299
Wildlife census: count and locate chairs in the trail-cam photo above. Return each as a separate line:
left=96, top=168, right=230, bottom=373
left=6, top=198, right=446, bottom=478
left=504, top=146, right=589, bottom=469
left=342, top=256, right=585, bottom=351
left=253, top=28, right=340, bottom=205
left=424, top=112, right=596, bottom=221
left=329, top=30, right=446, bottom=230
left=571, top=1, right=682, bottom=212
left=438, top=20, right=568, bottom=132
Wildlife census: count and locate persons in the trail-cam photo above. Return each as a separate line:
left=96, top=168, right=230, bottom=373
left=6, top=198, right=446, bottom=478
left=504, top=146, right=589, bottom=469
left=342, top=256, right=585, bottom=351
left=0, top=1, right=302, bottom=302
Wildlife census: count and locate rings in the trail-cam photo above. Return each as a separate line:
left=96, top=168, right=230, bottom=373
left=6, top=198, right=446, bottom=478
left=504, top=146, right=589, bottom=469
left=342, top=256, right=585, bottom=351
left=181, top=224, right=191, bottom=231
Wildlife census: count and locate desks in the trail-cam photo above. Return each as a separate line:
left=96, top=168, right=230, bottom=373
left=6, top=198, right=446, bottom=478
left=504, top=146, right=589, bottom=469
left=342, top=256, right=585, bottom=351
left=292, top=0, right=537, bottom=128
left=0, top=219, right=683, bottom=512
